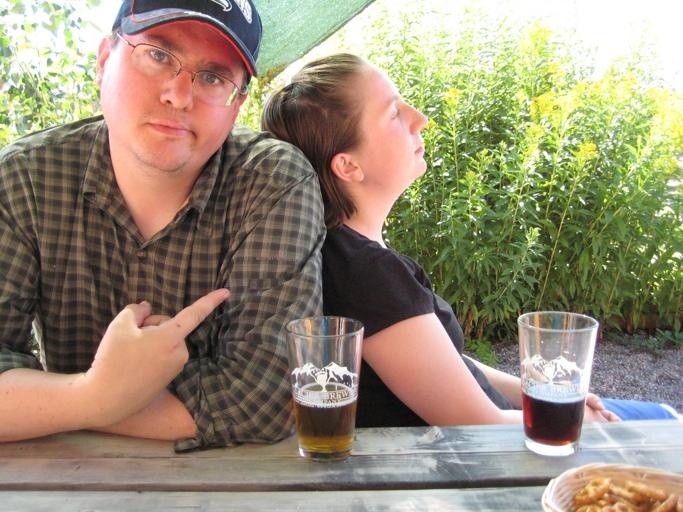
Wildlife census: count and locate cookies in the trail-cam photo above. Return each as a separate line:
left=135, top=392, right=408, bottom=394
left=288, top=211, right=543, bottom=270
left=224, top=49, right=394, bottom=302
left=573, top=478, right=682, bottom=512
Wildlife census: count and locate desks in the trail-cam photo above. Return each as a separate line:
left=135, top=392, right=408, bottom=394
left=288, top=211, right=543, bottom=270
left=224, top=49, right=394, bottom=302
left=0, top=418, right=683, bottom=512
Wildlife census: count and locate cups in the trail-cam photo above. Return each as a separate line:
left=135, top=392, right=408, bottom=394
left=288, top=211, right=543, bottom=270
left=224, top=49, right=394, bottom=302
left=284, top=314, right=366, bottom=464
left=516, top=310, right=601, bottom=459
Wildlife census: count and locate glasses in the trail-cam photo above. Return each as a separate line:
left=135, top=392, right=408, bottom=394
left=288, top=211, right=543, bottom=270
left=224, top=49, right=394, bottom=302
left=117, top=33, right=248, bottom=106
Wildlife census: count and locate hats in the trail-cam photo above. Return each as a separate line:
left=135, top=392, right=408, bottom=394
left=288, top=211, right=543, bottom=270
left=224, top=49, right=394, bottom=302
left=112, top=0, right=262, bottom=79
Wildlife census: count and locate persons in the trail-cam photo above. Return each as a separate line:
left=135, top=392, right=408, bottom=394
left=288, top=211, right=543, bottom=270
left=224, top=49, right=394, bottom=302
left=260, top=53, right=676, bottom=438
left=0, top=0, right=324, bottom=447
